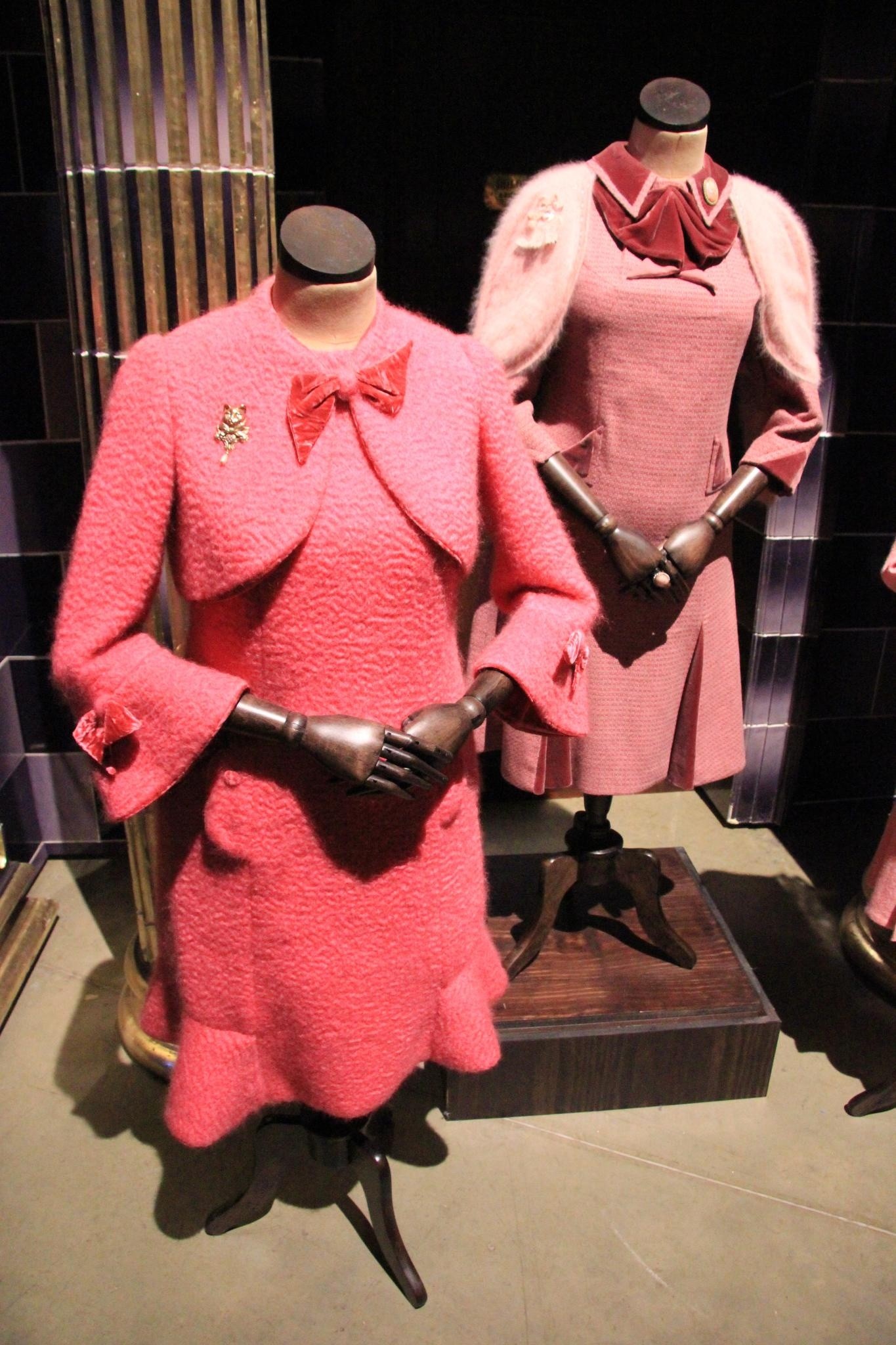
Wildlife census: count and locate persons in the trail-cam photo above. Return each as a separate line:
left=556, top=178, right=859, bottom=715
left=49, top=206, right=598, bottom=1148
left=470, top=78, right=820, bottom=795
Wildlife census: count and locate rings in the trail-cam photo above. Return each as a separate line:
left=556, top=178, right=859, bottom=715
left=654, top=573, right=670, bottom=588
